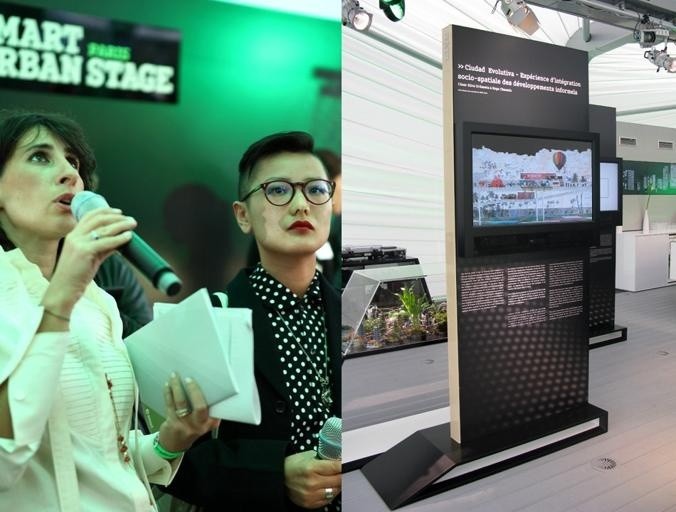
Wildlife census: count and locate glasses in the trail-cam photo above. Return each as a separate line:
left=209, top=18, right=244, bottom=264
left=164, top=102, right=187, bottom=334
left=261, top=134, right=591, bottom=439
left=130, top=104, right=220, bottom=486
left=240, top=178, right=336, bottom=205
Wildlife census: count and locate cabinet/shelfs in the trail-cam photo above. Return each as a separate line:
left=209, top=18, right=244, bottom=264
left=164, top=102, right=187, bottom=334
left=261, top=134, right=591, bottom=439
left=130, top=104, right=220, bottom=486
left=616, top=235, right=668, bottom=292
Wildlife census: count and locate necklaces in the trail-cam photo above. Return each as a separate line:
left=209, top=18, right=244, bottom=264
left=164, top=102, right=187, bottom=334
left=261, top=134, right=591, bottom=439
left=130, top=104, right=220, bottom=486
left=272, top=302, right=334, bottom=408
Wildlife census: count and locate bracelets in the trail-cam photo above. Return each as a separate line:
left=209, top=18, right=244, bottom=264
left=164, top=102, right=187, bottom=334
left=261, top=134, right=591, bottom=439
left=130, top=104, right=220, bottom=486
left=151, top=432, right=189, bottom=461
left=38, top=301, right=72, bottom=324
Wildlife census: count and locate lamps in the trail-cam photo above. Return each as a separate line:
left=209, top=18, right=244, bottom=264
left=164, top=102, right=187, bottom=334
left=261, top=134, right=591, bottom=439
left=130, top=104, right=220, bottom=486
left=342, top=0, right=407, bottom=32
left=627, top=9, right=676, bottom=77
left=490, top=0, right=542, bottom=38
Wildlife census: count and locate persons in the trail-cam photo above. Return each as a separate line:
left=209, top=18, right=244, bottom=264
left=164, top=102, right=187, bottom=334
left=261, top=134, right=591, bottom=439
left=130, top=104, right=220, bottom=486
left=242, top=145, right=341, bottom=286
left=0, top=108, right=225, bottom=512
left=143, top=131, right=342, bottom=510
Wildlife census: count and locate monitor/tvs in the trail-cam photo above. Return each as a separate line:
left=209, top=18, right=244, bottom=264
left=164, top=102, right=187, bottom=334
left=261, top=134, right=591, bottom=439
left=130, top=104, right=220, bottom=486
left=598, top=158, right=624, bottom=226
left=458, top=121, right=601, bottom=258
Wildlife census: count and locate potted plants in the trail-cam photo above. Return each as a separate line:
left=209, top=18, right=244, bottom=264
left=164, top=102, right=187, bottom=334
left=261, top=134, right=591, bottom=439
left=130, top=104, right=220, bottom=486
left=642, top=182, right=653, bottom=234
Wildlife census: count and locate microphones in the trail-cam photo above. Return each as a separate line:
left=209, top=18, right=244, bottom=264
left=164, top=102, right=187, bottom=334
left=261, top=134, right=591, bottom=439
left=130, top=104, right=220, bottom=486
left=70, top=189, right=184, bottom=297
left=313, top=416, right=342, bottom=460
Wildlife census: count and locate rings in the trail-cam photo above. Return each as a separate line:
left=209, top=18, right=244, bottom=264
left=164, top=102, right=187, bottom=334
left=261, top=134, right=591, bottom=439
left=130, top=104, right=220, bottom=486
left=323, top=486, right=334, bottom=501
left=172, top=407, right=192, bottom=417
left=90, top=229, right=102, bottom=243
